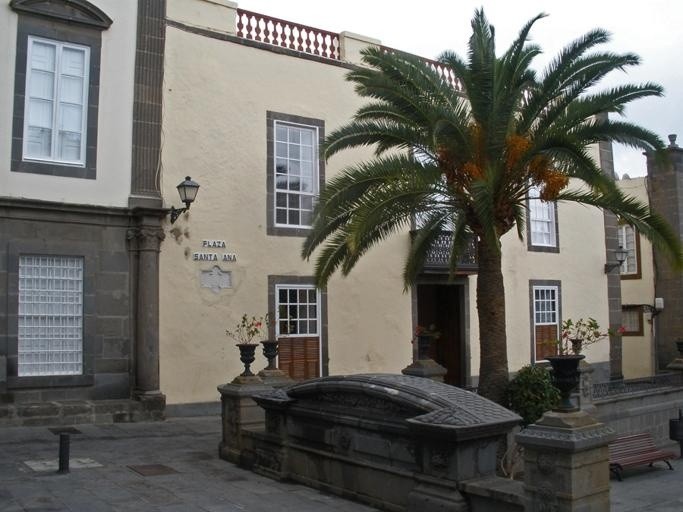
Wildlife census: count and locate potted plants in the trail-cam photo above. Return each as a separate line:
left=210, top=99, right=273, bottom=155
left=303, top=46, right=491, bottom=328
left=226, top=308, right=295, bottom=377
left=411, top=323, right=440, bottom=359
left=537, top=316, right=625, bottom=412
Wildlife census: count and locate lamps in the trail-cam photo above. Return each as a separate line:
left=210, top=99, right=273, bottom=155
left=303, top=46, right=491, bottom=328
left=604, top=245, right=628, bottom=275
left=164, top=175, right=201, bottom=223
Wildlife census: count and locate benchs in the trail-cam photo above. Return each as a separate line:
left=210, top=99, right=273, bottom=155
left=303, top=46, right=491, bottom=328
left=607, top=431, right=675, bottom=481
